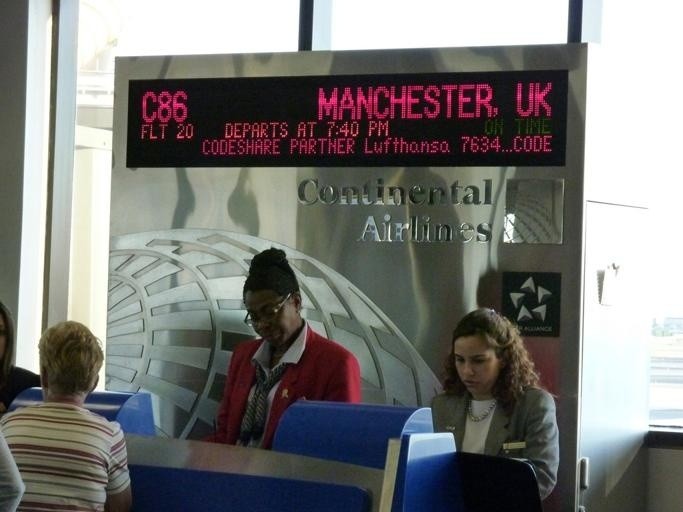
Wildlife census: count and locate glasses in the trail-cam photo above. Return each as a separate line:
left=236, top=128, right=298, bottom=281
left=244, top=293, right=290, bottom=327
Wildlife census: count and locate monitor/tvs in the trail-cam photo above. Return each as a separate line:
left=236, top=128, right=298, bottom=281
left=7, top=388, right=156, bottom=433
left=271, top=398, right=435, bottom=468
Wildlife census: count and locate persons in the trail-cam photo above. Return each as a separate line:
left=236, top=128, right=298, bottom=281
left=0, top=320, right=133, bottom=512
left=183, top=247, right=362, bottom=475
left=0, top=300, right=41, bottom=419
left=427, top=307, right=559, bottom=502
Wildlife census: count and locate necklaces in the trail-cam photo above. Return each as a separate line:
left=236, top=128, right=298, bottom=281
left=271, top=351, right=283, bottom=358
left=467, top=399, right=496, bottom=422
